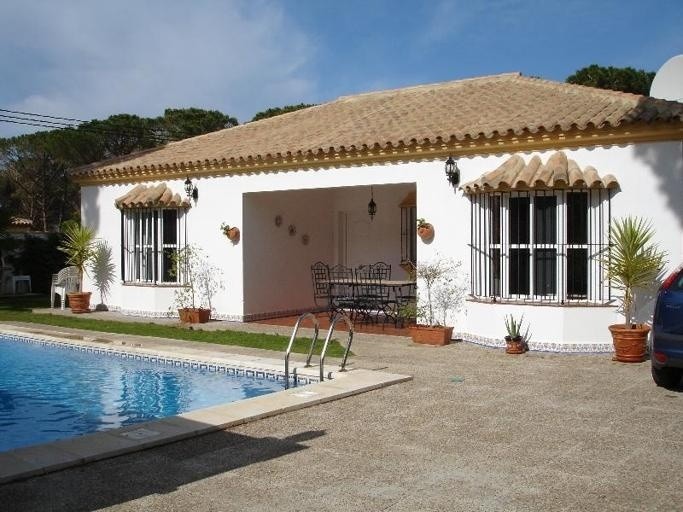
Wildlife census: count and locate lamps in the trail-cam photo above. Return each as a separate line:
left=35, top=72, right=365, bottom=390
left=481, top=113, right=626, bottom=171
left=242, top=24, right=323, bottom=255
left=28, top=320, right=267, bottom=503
left=367, top=192, right=377, bottom=224
left=443, top=155, right=458, bottom=185
left=183, top=176, right=197, bottom=199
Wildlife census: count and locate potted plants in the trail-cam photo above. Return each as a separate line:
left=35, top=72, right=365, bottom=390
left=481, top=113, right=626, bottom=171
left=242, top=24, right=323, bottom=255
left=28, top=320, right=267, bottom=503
left=59, top=224, right=101, bottom=314
left=414, top=217, right=433, bottom=240
left=161, top=242, right=211, bottom=323
left=503, top=313, right=525, bottom=354
left=218, top=221, right=239, bottom=242
left=589, top=214, right=670, bottom=363
left=395, top=251, right=462, bottom=346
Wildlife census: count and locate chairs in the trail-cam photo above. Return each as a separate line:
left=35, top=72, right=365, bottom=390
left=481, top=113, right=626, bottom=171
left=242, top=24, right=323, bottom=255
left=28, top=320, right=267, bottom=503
left=310, top=260, right=416, bottom=331
left=49, top=264, right=80, bottom=311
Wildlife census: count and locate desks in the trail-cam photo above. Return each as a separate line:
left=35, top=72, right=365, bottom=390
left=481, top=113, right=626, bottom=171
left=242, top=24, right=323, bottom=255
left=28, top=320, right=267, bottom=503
left=8, top=275, right=31, bottom=295
